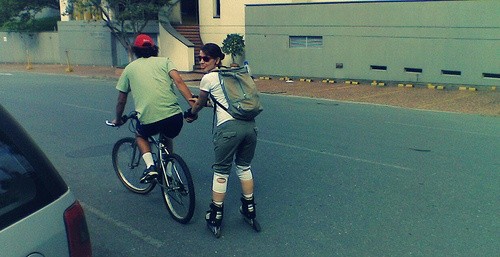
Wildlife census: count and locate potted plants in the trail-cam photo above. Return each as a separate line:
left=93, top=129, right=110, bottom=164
left=220, top=33, right=245, bottom=67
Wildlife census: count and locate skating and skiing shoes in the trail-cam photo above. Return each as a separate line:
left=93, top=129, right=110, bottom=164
left=204, top=198, right=224, bottom=237
left=238, top=197, right=261, bottom=232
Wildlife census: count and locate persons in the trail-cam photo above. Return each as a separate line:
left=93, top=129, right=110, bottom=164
left=183, top=43, right=258, bottom=225
left=111, top=35, right=199, bottom=182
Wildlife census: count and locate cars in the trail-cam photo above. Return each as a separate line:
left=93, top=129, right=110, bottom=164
left=0, top=103, right=93, bottom=257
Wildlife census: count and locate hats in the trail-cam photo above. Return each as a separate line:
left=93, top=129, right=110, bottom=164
left=134, top=34, right=154, bottom=49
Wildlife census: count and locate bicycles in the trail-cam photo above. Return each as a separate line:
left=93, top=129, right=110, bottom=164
left=105, top=95, right=195, bottom=224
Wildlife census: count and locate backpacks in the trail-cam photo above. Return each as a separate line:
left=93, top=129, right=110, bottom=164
left=209, top=62, right=263, bottom=134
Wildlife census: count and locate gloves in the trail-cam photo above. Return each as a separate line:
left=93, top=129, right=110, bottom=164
left=184, top=107, right=199, bottom=123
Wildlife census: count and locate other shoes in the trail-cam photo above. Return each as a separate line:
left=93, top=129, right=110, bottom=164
left=140, top=165, right=159, bottom=184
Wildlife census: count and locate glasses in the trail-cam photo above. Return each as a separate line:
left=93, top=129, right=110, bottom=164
left=198, top=55, right=213, bottom=62
